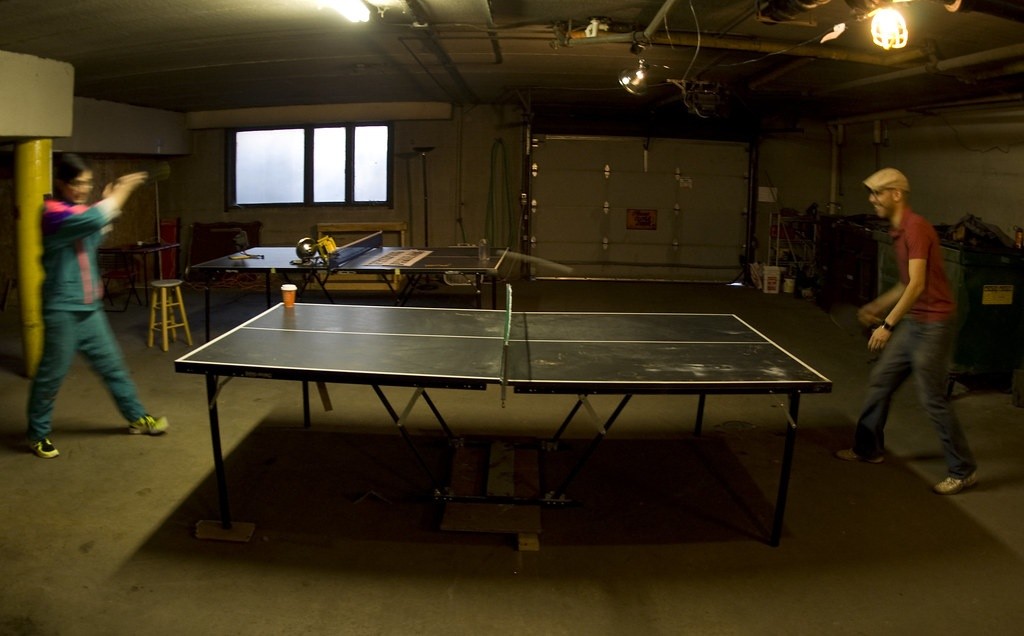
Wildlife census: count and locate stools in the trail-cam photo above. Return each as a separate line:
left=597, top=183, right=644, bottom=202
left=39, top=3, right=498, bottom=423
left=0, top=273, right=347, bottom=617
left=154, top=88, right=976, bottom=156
left=148, top=279, right=194, bottom=353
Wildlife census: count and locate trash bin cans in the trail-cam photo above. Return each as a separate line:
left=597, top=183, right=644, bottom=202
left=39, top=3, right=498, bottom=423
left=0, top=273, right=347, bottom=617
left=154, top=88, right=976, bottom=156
left=872, top=224, right=1024, bottom=386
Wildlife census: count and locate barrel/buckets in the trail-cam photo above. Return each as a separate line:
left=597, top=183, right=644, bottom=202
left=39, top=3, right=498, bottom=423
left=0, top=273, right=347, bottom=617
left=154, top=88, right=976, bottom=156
left=763, top=270, right=780, bottom=293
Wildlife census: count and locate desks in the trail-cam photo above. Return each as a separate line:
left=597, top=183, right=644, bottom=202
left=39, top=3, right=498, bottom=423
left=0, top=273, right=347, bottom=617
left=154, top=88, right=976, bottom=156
left=99, top=243, right=181, bottom=307
left=191, top=229, right=510, bottom=342
left=173, top=283, right=836, bottom=549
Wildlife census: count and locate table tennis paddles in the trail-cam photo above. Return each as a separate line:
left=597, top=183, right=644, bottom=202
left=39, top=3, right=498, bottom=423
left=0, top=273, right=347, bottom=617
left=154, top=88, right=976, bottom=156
left=829, top=302, right=884, bottom=335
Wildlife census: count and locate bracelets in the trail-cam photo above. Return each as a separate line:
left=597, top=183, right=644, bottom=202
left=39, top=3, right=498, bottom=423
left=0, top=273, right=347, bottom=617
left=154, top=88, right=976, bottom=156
left=880, top=320, right=895, bottom=333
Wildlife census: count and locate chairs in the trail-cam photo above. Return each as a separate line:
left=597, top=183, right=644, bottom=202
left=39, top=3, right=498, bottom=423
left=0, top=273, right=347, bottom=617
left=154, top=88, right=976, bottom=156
left=96, top=247, right=142, bottom=313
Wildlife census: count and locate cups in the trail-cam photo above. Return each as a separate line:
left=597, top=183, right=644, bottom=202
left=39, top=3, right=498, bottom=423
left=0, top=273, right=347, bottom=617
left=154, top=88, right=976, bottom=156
left=281, top=284, right=297, bottom=308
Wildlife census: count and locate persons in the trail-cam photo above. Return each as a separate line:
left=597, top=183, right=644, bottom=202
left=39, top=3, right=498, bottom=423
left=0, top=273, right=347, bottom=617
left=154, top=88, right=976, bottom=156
left=836, top=167, right=982, bottom=495
left=25, top=154, right=169, bottom=459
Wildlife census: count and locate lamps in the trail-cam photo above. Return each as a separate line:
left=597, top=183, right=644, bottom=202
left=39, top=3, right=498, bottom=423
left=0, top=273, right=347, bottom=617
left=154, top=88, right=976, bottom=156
left=615, top=45, right=654, bottom=98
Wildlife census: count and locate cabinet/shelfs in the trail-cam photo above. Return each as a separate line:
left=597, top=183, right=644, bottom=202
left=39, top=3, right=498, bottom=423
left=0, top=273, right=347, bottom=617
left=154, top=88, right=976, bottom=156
left=860, top=226, right=1015, bottom=401
left=766, top=211, right=818, bottom=278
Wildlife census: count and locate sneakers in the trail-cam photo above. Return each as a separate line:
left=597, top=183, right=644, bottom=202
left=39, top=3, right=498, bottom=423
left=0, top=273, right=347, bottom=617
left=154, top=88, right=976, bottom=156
left=27, top=437, right=60, bottom=459
left=128, top=415, right=169, bottom=434
left=835, top=447, right=884, bottom=464
left=933, top=471, right=977, bottom=495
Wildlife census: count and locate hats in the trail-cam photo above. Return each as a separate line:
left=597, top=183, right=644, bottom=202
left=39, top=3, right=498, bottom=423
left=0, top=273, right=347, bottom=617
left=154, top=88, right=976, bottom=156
left=862, top=167, right=910, bottom=192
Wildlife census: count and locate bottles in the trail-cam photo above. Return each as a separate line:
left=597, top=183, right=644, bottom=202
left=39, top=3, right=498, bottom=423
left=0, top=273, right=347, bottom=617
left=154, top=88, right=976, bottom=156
left=1013, top=225, right=1023, bottom=249
left=479, top=239, right=490, bottom=260
